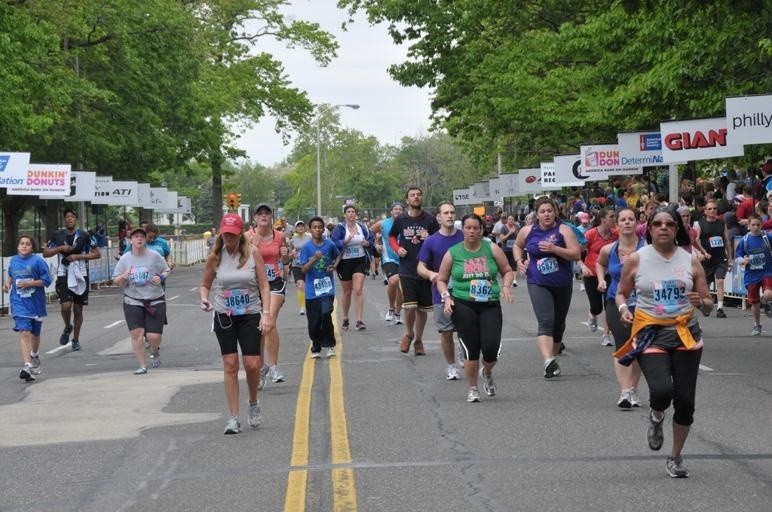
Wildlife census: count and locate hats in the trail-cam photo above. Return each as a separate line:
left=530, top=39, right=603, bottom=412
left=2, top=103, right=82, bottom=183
left=295, top=220, right=304, bottom=227
left=62, top=209, right=80, bottom=215
left=582, top=213, right=589, bottom=224
left=129, top=228, right=146, bottom=237
left=575, top=211, right=583, bottom=217
left=220, top=213, right=245, bottom=236
left=253, top=203, right=273, bottom=214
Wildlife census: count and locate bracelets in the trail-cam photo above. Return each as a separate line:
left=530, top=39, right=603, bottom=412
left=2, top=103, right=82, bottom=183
left=515, top=257, right=524, bottom=264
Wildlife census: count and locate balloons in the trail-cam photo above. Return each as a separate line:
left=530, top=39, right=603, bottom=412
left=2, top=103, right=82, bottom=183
left=224, top=191, right=241, bottom=210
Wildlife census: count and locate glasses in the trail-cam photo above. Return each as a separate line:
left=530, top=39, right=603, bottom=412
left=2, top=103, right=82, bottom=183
left=679, top=214, right=689, bottom=218
left=708, top=208, right=716, bottom=211
left=649, top=219, right=676, bottom=228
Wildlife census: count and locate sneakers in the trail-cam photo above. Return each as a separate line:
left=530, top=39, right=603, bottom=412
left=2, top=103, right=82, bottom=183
left=414, top=341, right=426, bottom=356
left=325, top=346, right=337, bottom=358
left=446, top=364, right=459, bottom=380
left=355, top=320, right=366, bottom=331
left=341, top=318, right=350, bottom=331
left=513, top=280, right=518, bottom=287
left=224, top=415, right=243, bottom=435
left=270, top=365, right=283, bottom=383
left=466, top=384, right=482, bottom=402
left=257, top=364, right=270, bottom=391
left=385, top=310, right=394, bottom=321
left=133, top=366, right=148, bottom=375
left=716, top=308, right=727, bottom=319
left=630, top=388, right=646, bottom=410
left=71, top=338, right=80, bottom=351
left=270, top=366, right=284, bottom=383
left=479, top=366, right=497, bottom=396
left=311, top=349, right=322, bottom=359
left=247, top=403, right=262, bottom=428
left=20, top=365, right=35, bottom=382
left=617, top=389, right=632, bottom=410
left=59, top=324, right=73, bottom=345
left=588, top=310, right=598, bottom=332
left=666, top=455, right=691, bottom=480
left=151, top=352, right=161, bottom=369
left=543, top=359, right=561, bottom=379
left=760, top=296, right=772, bottom=318
left=646, top=408, right=666, bottom=451
left=750, top=325, right=763, bottom=337
left=456, top=352, right=467, bottom=367
left=601, top=334, right=614, bottom=347
left=30, top=355, right=42, bottom=375
left=400, top=331, right=415, bottom=354
left=393, top=313, right=403, bottom=325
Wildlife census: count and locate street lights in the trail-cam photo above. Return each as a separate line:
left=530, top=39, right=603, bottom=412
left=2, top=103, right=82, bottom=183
left=317, top=104, right=359, bottom=216
left=314, top=101, right=362, bottom=222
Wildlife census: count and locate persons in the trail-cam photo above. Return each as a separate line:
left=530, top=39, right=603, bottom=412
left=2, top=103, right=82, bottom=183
left=735, top=212, right=772, bottom=336
left=280, top=216, right=295, bottom=237
left=589, top=201, right=601, bottom=226
left=0, top=234, right=50, bottom=382
left=588, top=166, right=658, bottom=205
left=371, top=203, right=404, bottom=326
left=488, top=212, right=508, bottom=241
left=576, top=207, right=620, bottom=347
left=375, top=211, right=388, bottom=284
left=205, top=226, right=218, bottom=253
left=559, top=194, right=569, bottom=203
left=417, top=203, right=466, bottom=381
left=43, top=207, right=101, bottom=350
left=478, top=221, right=491, bottom=242
left=300, top=217, right=340, bottom=358
left=616, top=206, right=714, bottom=477
left=692, top=200, right=734, bottom=318
left=595, top=208, right=649, bottom=407
left=361, top=216, right=369, bottom=225
left=637, top=201, right=660, bottom=238
left=111, top=229, right=170, bottom=375
left=525, top=213, right=536, bottom=226
left=368, top=248, right=380, bottom=278
left=323, top=223, right=334, bottom=239
left=332, top=206, right=374, bottom=332
left=199, top=214, right=271, bottom=434
left=141, top=224, right=174, bottom=350
left=500, top=215, right=520, bottom=287
left=553, top=199, right=586, bottom=353
left=289, top=220, right=313, bottom=315
left=550, top=191, right=561, bottom=200
left=282, top=263, right=292, bottom=290
left=250, top=204, right=290, bottom=390
left=676, top=206, right=711, bottom=262
left=571, top=192, right=581, bottom=205
left=439, top=213, right=514, bottom=402
left=140, top=220, right=151, bottom=234
left=679, top=165, right=771, bottom=228
left=387, top=187, right=440, bottom=355
left=118, top=219, right=133, bottom=254
left=513, top=196, right=580, bottom=378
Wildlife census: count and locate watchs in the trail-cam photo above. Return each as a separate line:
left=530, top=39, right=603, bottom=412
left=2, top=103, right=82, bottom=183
left=441, top=290, right=448, bottom=304
left=697, top=297, right=704, bottom=311
